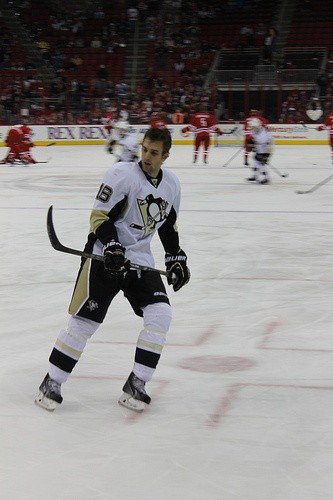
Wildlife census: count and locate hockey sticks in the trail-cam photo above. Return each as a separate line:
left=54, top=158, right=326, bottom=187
left=222, top=145, right=244, bottom=168
left=295, top=170, right=333, bottom=195
left=35, top=142, right=56, bottom=148
left=46, top=203, right=169, bottom=281
left=209, top=125, right=238, bottom=136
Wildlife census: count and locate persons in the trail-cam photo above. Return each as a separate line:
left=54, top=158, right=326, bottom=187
left=35, top=126, right=191, bottom=411
left=0, top=0, right=333, bottom=183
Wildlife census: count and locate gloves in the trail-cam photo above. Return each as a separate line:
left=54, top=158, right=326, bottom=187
left=162, top=250, right=189, bottom=292
left=101, top=243, right=124, bottom=275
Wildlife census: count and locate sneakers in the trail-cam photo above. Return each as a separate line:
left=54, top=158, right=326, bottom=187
left=243, top=162, right=250, bottom=166
left=34, top=372, right=63, bottom=411
left=0, top=159, right=34, bottom=166
left=119, top=370, right=151, bottom=411
left=244, top=176, right=268, bottom=185
left=192, top=159, right=197, bottom=163
left=204, top=160, right=207, bottom=163
